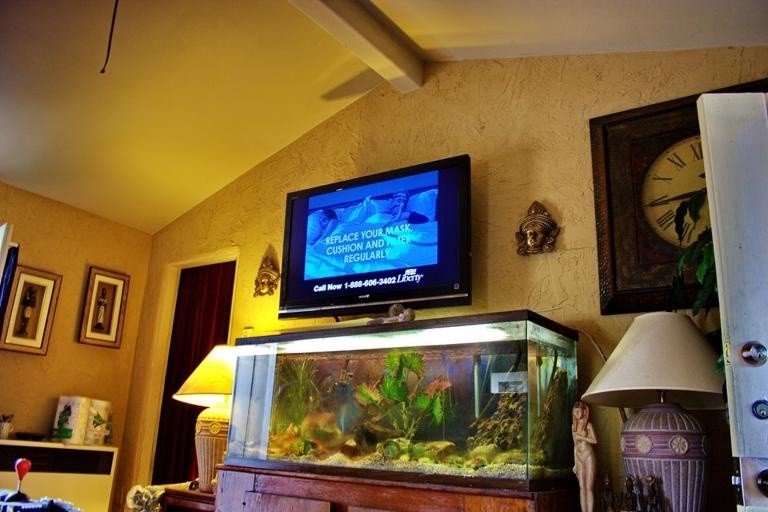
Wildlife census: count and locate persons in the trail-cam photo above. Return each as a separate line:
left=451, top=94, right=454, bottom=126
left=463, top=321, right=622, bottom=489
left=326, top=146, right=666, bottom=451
left=571, top=401, right=598, bottom=512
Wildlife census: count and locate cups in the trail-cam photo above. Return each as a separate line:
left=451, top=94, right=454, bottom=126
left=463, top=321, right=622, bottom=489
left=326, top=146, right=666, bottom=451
left=0, top=421, right=16, bottom=439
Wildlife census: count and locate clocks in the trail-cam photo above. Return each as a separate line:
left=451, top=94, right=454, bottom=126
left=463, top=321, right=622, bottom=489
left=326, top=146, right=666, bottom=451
left=589, top=78, right=764, bottom=314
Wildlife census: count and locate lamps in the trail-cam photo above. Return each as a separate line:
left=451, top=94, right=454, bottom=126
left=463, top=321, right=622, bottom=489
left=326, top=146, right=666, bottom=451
left=172, top=345, right=238, bottom=494
left=582, top=311, right=728, bottom=511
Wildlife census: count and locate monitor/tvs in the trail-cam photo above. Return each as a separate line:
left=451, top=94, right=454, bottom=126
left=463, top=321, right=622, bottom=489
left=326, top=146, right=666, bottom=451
left=277, top=153, right=473, bottom=320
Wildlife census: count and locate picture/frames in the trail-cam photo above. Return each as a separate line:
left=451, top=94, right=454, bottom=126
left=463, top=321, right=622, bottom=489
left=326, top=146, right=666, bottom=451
left=1, top=266, right=63, bottom=357
left=78, top=266, right=131, bottom=350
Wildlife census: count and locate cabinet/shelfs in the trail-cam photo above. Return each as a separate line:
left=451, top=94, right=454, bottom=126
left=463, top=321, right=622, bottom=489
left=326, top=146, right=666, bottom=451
left=213, top=465, right=578, bottom=512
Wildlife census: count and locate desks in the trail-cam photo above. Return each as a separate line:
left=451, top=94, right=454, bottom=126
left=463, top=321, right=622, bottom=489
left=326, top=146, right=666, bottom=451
left=160, top=488, right=216, bottom=512
